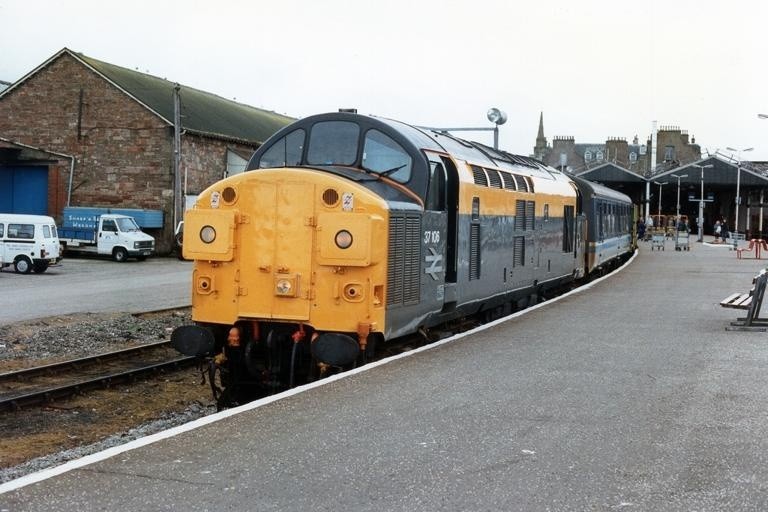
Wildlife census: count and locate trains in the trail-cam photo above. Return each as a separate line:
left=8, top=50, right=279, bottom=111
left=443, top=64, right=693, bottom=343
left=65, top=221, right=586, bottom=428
left=162, top=103, right=641, bottom=416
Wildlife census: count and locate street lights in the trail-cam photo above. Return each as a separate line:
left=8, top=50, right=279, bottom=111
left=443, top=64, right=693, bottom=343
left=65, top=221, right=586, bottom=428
left=724, top=146, right=755, bottom=250
left=688, top=163, right=715, bottom=245
left=669, top=173, right=690, bottom=238
left=653, top=181, right=670, bottom=230
left=758, top=113, right=768, bottom=119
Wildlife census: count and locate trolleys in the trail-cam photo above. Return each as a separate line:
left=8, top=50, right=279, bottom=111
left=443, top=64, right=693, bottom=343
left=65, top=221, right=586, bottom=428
left=672, top=229, right=693, bottom=254
left=649, top=231, right=665, bottom=252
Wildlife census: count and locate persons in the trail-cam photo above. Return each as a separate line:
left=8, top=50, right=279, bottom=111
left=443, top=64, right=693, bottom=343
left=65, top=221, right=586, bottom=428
left=637, top=216, right=646, bottom=242
left=713, top=220, right=720, bottom=242
left=635, top=215, right=642, bottom=234
left=645, top=215, right=654, bottom=226
left=721, top=217, right=729, bottom=245
left=678, top=213, right=691, bottom=251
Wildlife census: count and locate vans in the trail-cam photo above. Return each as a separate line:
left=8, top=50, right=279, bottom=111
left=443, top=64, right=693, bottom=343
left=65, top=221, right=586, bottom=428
left=0, top=212, right=60, bottom=275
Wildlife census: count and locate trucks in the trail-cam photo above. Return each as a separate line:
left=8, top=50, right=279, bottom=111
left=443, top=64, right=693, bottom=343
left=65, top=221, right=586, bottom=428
left=51, top=212, right=157, bottom=264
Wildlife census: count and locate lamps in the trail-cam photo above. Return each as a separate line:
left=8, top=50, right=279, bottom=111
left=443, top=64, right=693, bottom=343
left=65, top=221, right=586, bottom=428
left=486, top=107, right=508, bottom=125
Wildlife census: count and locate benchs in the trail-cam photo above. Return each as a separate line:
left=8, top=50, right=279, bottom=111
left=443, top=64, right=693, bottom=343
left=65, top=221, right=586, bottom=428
left=720, top=268, right=768, bottom=332
